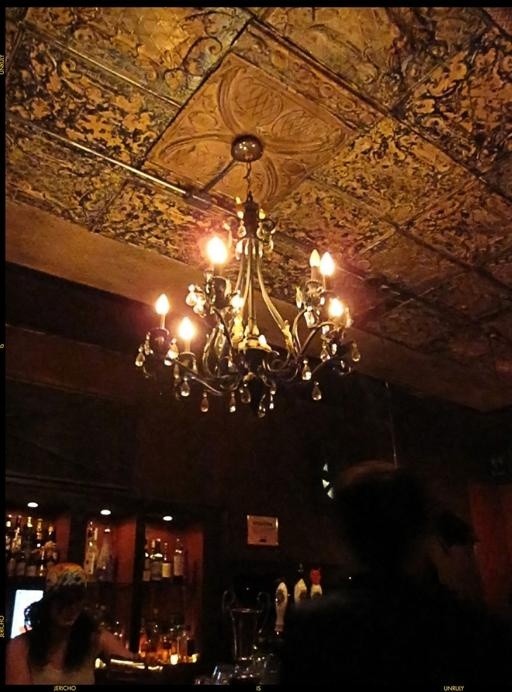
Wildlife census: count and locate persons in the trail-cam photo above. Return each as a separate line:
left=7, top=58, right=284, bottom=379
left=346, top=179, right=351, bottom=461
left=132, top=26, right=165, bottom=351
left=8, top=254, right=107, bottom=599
left=437, top=515, right=480, bottom=586
left=6, top=563, right=135, bottom=685
left=277, top=460, right=511, bottom=691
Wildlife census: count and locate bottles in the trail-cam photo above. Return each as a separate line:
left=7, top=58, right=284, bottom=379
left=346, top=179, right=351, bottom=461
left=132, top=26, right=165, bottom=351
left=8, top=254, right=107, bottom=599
left=4, top=513, right=60, bottom=577
left=198, top=648, right=279, bottom=685
left=142, top=535, right=186, bottom=582
left=94, top=603, right=195, bottom=668
left=85, top=523, right=116, bottom=581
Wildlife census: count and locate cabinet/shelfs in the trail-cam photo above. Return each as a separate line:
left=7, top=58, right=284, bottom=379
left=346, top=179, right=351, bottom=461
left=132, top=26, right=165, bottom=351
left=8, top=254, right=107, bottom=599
left=1, top=475, right=213, bottom=684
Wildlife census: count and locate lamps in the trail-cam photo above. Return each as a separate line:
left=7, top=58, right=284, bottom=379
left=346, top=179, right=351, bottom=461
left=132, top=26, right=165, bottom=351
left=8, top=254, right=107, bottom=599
left=134, top=133, right=362, bottom=421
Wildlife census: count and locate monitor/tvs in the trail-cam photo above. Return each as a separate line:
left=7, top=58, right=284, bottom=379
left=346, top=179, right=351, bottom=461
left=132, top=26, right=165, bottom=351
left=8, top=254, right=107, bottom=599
left=6, top=585, right=45, bottom=640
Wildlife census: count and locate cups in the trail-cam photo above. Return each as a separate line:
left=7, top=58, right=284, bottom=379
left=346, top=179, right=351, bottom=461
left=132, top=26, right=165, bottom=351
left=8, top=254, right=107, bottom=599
left=231, top=608, right=260, bottom=664
